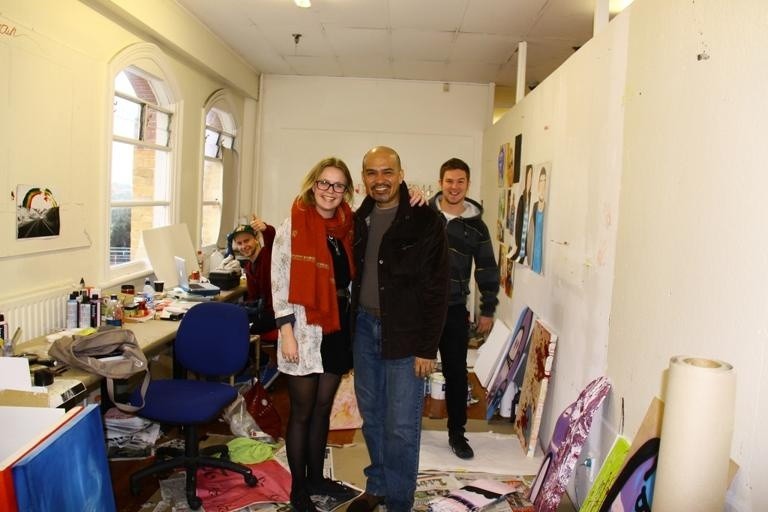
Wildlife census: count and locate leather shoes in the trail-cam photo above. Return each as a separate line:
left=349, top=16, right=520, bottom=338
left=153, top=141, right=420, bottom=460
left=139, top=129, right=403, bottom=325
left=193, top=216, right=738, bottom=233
left=288, top=493, right=317, bottom=512
left=308, top=478, right=352, bottom=496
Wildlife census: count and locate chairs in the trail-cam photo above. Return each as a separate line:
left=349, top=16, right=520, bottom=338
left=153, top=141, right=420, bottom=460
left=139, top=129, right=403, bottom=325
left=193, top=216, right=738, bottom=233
left=129, top=302, right=258, bottom=509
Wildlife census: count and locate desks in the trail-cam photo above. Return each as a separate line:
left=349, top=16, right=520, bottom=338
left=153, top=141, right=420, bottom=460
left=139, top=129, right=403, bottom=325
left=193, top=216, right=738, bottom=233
left=11, top=274, right=247, bottom=403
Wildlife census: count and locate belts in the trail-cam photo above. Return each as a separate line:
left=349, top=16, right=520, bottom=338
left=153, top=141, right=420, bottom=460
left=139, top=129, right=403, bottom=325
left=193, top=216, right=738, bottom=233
left=360, top=306, right=381, bottom=316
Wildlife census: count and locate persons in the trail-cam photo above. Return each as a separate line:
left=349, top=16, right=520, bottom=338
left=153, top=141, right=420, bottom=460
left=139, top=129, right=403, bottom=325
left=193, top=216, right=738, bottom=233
left=427, top=158, right=501, bottom=460
left=509, top=165, right=533, bottom=265
left=526, top=167, right=548, bottom=276
left=270, top=159, right=354, bottom=512
left=220, top=213, right=280, bottom=386
left=345, top=146, right=446, bottom=512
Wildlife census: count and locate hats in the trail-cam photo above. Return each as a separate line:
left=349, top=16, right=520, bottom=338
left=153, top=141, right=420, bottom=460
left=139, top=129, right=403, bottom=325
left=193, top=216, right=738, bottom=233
left=228, top=224, right=257, bottom=239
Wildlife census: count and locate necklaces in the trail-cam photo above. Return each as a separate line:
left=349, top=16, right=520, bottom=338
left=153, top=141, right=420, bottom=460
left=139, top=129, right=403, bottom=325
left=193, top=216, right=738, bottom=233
left=328, top=238, right=341, bottom=256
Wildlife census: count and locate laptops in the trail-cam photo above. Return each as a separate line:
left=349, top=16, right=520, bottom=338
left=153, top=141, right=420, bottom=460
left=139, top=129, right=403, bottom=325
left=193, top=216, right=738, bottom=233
left=175, top=255, right=222, bottom=295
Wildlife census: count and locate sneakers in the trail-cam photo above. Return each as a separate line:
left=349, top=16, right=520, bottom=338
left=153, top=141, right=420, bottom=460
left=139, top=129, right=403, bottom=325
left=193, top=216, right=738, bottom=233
left=449, top=438, right=473, bottom=458
left=347, top=493, right=385, bottom=510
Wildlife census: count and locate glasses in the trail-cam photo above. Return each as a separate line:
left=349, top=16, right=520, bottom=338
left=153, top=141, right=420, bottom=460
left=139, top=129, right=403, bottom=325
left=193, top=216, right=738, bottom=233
left=315, top=180, right=349, bottom=193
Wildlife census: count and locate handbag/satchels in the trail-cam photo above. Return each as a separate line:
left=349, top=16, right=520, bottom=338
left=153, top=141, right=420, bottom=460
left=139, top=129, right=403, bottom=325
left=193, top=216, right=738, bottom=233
left=47, top=326, right=151, bottom=413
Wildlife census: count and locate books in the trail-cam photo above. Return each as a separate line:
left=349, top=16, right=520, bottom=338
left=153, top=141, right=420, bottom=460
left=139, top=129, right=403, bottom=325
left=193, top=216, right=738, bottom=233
left=103, top=408, right=161, bottom=444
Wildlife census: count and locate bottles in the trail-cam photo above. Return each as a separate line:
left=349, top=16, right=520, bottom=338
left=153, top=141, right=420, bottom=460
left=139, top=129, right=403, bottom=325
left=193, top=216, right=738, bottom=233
left=0, top=314, right=9, bottom=346
left=80, top=296, right=91, bottom=329
left=90, top=294, right=101, bottom=328
left=143, top=280, right=154, bottom=311
left=66, top=294, right=79, bottom=328
left=197, top=251, right=205, bottom=272
left=107, top=295, right=123, bottom=329
left=192, top=271, right=200, bottom=282
left=73, top=291, right=81, bottom=300
